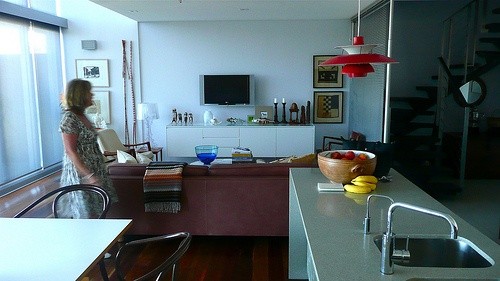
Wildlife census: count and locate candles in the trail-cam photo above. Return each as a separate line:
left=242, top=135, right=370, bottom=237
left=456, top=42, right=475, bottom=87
left=273, top=98, right=277, bottom=103
left=282, top=97, right=285, bottom=103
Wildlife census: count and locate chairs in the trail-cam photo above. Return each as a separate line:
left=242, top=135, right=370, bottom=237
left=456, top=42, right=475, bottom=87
left=96, top=129, right=151, bottom=158
left=14, top=184, right=109, bottom=281
left=116, top=232, right=191, bottom=281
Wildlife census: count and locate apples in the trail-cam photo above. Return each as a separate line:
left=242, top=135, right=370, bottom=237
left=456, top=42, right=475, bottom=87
left=331, top=151, right=367, bottom=160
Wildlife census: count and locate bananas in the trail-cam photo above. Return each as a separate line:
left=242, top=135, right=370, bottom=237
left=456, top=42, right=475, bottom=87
left=344, top=175, right=378, bottom=194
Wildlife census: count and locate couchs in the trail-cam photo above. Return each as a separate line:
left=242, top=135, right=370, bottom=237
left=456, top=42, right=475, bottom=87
left=107, top=163, right=312, bottom=236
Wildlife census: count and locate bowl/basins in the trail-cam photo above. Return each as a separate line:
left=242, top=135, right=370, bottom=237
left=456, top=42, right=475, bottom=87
left=318, top=150, right=377, bottom=184
left=195, top=145, right=218, bottom=164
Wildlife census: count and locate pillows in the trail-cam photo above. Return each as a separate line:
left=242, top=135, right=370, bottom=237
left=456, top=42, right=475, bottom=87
left=117, top=150, right=152, bottom=163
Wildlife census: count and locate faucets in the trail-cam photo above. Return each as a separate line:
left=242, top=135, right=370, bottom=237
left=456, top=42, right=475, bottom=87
left=363, top=195, right=394, bottom=233
left=380, top=202, right=459, bottom=275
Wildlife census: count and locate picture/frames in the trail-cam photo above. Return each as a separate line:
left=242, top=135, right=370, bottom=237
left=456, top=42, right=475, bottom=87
left=84, top=91, right=110, bottom=124
left=313, top=55, right=343, bottom=88
left=313, top=91, right=343, bottom=123
left=255, top=106, right=273, bottom=120
left=76, top=59, right=109, bottom=87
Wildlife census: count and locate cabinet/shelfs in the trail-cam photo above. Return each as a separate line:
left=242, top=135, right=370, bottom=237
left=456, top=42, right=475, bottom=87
left=165, top=126, right=315, bottom=157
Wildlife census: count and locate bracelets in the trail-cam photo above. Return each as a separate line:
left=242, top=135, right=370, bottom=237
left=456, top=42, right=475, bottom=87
left=87, top=172, right=95, bottom=180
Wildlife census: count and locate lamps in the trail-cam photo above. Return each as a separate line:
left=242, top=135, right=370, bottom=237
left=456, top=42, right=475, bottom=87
left=319, top=0, right=400, bottom=78
left=137, top=104, right=160, bottom=149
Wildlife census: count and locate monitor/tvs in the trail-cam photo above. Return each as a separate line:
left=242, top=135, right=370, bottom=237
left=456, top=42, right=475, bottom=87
left=199, top=74, right=256, bottom=107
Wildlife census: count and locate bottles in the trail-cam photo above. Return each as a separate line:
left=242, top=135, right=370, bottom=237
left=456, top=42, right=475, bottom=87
left=171, top=109, right=193, bottom=126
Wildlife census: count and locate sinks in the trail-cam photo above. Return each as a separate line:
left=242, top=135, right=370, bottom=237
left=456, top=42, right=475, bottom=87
left=372, top=234, right=495, bottom=268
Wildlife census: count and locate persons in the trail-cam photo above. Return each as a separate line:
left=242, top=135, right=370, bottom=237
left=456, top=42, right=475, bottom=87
left=58, top=78, right=115, bottom=259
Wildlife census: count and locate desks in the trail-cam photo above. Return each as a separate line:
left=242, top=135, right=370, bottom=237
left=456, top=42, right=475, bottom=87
left=137, top=147, right=163, bottom=162
left=0, top=218, right=133, bottom=281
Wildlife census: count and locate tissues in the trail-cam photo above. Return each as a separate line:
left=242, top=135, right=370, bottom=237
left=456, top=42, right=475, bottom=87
left=232, top=148, right=253, bottom=161
left=135, top=143, right=148, bottom=152
